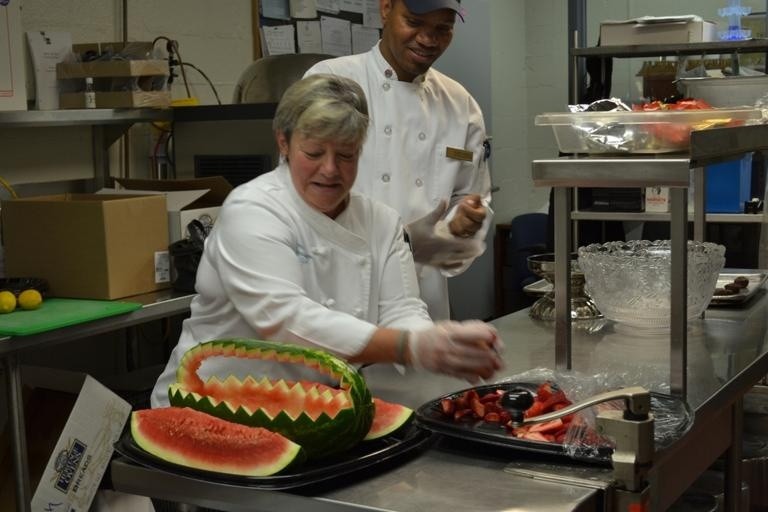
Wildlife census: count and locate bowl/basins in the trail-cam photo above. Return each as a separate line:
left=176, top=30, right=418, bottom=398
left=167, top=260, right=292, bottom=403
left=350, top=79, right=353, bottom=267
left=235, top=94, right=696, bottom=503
left=574, top=237, right=727, bottom=330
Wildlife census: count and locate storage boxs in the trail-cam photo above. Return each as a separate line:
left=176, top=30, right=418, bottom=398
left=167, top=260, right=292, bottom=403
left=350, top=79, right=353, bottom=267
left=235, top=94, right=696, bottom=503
left=0, top=177, right=237, bottom=299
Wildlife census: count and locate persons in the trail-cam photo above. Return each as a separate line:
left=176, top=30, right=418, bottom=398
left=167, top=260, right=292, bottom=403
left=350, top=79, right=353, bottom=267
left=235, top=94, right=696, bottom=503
left=149, top=70, right=506, bottom=420
left=298, top=2, right=497, bottom=325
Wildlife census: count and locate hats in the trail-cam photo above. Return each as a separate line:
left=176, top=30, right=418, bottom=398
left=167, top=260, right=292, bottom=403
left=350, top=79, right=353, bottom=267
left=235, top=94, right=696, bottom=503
left=403, top=0, right=465, bottom=23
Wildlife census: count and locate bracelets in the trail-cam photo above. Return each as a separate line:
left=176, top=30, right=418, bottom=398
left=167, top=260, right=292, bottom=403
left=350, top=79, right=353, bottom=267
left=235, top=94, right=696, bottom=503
left=392, top=330, right=412, bottom=366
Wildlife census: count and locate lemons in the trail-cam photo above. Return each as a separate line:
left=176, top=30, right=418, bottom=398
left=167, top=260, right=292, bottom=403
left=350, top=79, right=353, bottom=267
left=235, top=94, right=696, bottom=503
left=0, top=291, right=17, bottom=314
left=18, top=289, right=42, bottom=310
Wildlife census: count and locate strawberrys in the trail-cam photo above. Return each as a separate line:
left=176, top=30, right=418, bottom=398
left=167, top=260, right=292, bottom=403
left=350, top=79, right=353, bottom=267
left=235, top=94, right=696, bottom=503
left=439, top=380, right=601, bottom=447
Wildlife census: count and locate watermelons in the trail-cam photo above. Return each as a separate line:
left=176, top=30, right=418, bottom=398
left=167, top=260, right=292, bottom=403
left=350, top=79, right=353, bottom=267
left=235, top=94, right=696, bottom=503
left=130, top=339, right=415, bottom=478
